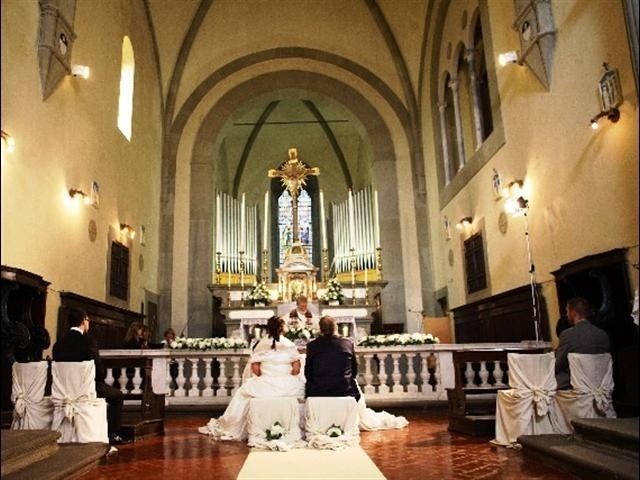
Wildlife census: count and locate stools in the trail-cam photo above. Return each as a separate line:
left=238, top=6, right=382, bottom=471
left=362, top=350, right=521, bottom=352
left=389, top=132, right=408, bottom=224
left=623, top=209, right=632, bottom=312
left=303, top=394, right=363, bottom=451
left=245, top=397, right=307, bottom=452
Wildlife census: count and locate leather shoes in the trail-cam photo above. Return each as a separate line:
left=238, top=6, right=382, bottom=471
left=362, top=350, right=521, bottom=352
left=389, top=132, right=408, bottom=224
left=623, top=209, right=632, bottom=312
left=108, top=435, right=131, bottom=445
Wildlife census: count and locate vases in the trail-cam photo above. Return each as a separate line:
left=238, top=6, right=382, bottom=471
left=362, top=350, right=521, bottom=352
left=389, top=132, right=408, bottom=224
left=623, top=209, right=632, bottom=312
left=254, top=300, right=266, bottom=309
left=328, top=300, right=340, bottom=308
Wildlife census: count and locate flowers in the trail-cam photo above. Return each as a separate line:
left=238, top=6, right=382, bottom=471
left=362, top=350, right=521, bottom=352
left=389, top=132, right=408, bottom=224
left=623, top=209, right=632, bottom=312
left=264, top=421, right=286, bottom=441
left=324, top=423, right=346, bottom=438
left=245, top=277, right=274, bottom=307
left=171, top=329, right=442, bottom=351
left=324, top=279, right=345, bottom=304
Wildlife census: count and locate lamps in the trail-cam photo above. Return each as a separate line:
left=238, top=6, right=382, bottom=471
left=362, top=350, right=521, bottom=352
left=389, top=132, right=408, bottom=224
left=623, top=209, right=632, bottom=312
left=497, top=49, right=519, bottom=69
left=68, top=187, right=93, bottom=207
left=454, top=215, right=476, bottom=230
left=590, top=108, right=622, bottom=131
left=71, top=63, right=90, bottom=81
left=120, top=223, right=137, bottom=241
left=0, top=128, right=17, bottom=157
left=493, top=179, right=531, bottom=218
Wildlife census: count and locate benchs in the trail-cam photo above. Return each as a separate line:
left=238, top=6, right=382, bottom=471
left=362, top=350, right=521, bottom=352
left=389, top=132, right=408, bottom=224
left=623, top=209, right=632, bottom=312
left=98, top=354, right=170, bottom=446
left=443, top=347, right=554, bottom=437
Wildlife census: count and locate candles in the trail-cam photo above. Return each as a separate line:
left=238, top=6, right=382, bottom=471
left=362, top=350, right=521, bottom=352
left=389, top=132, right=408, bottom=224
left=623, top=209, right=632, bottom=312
left=318, top=187, right=382, bottom=288
left=212, top=189, right=270, bottom=288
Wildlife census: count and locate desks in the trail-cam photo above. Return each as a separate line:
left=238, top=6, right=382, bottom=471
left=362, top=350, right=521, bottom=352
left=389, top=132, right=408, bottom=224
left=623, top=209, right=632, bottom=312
left=241, top=315, right=359, bottom=337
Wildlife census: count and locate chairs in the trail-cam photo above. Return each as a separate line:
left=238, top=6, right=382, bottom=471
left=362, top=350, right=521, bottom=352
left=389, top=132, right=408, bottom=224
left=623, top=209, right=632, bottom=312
left=489, top=348, right=569, bottom=455
left=557, top=351, right=620, bottom=435
left=9, top=359, right=56, bottom=433
left=48, top=358, right=109, bottom=446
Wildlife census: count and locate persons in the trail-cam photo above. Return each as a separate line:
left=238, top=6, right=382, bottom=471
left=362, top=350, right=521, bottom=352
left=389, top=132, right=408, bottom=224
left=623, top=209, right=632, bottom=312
left=289, top=295, right=312, bottom=325
left=121, top=321, right=143, bottom=349
left=555, top=296, right=612, bottom=391
left=304, top=316, right=358, bottom=396
left=161, top=327, right=179, bottom=343
left=143, top=325, right=165, bottom=349
left=51, top=306, right=132, bottom=446
left=249, top=315, right=301, bottom=397
